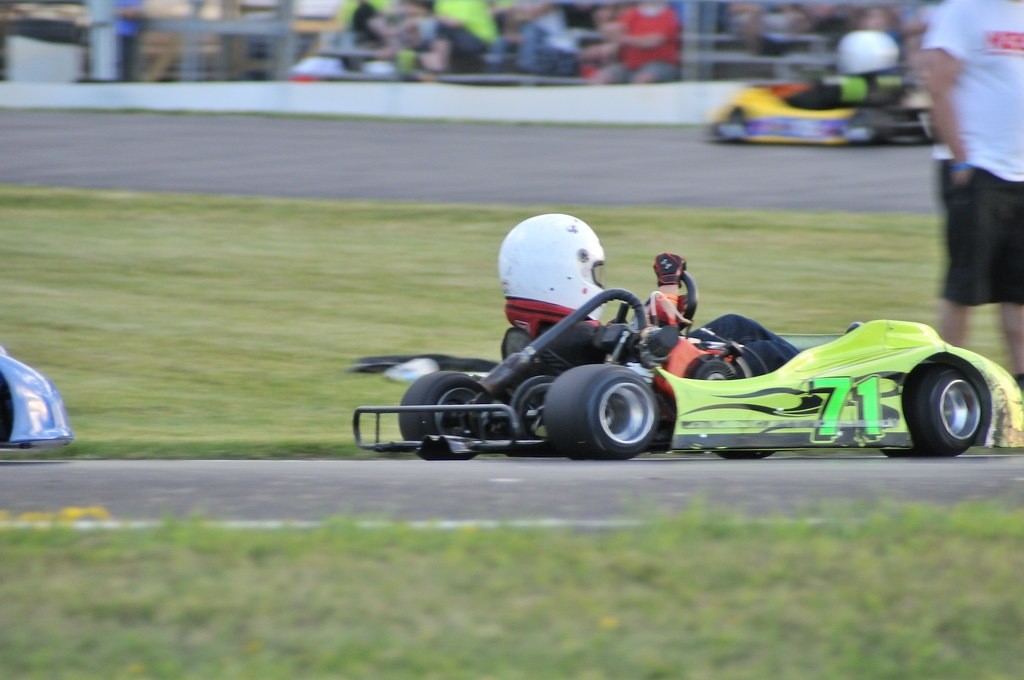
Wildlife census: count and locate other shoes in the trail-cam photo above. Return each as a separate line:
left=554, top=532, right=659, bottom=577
left=846, top=321, right=863, bottom=333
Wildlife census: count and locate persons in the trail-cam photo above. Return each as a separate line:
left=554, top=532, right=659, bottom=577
left=497, top=213, right=798, bottom=380
left=317, top=0, right=925, bottom=87
left=922, top=0, right=1024, bottom=392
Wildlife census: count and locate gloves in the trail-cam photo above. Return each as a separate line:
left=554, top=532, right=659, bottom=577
left=655, top=253, right=686, bottom=288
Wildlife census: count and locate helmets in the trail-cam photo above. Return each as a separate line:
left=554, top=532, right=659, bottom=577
left=836, top=28, right=901, bottom=76
left=500, top=212, right=606, bottom=321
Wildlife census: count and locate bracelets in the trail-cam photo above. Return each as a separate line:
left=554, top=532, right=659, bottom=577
left=950, top=162, right=971, bottom=171
